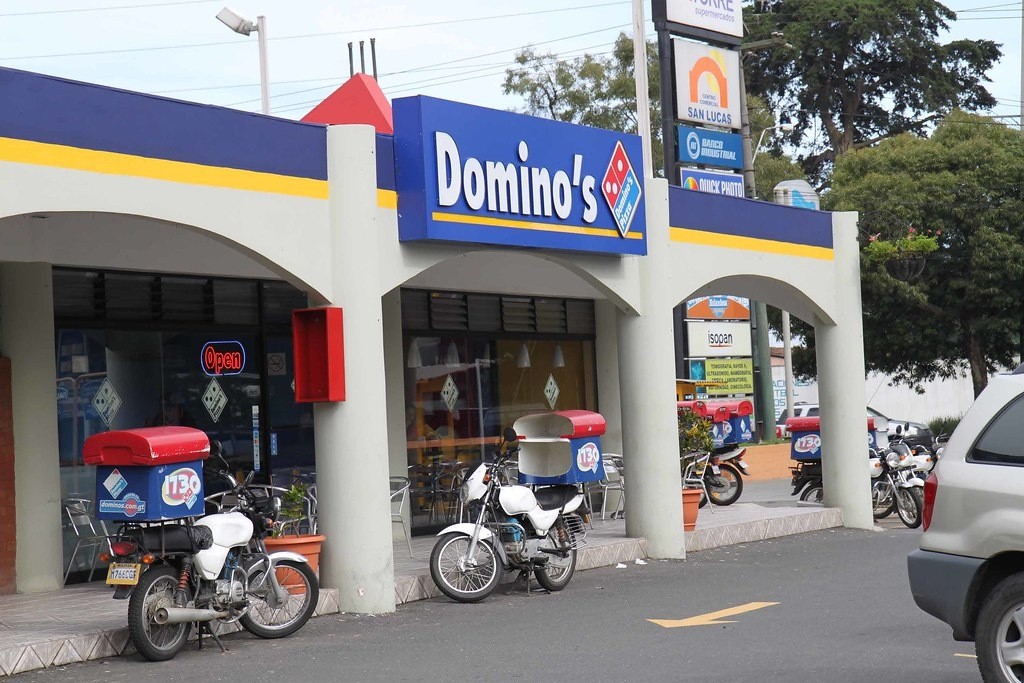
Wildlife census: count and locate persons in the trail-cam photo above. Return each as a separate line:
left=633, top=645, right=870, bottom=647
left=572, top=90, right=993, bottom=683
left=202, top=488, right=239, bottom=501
left=405, top=402, right=418, bottom=465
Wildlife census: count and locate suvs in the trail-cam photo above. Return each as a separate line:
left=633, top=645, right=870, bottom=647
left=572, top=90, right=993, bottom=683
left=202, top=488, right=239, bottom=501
left=777, top=402, right=936, bottom=449
left=907, top=360, right=1024, bottom=683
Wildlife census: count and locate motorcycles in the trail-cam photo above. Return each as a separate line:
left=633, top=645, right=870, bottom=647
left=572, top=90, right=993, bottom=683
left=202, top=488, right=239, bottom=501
left=681, top=439, right=751, bottom=510
left=107, top=438, right=321, bottom=662
left=429, top=426, right=594, bottom=602
left=787, top=420, right=950, bottom=529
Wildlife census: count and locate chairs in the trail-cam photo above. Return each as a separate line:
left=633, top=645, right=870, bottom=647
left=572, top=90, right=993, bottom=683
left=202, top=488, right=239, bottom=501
left=408, top=459, right=556, bottom=528
left=581, top=453, right=624, bottom=530
left=389, top=476, right=414, bottom=559
left=63, top=498, right=135, bottom=584
left=205, top=484, right=319, bottom=536
left=680, top=451, right=714, bottom=514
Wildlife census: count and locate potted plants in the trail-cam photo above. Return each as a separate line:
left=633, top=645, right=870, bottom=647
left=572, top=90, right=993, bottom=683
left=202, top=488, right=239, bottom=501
left=262, top=481, right=326, bottom=594
left=677, top=407, right=714, bottom=531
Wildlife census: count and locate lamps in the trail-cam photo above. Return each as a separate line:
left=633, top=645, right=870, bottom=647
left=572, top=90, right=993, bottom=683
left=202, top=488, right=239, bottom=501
left=553, top=341, right=565, bottom=369
left=514, top=339, right=531, bottom=368
left=446, top=339, right=460, bottom=365
left=484, top=342, right=491, bottom=367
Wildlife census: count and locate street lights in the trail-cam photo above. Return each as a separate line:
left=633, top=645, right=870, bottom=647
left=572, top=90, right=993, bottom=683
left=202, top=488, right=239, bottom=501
left=214, top=5, right=271, bottom=115
